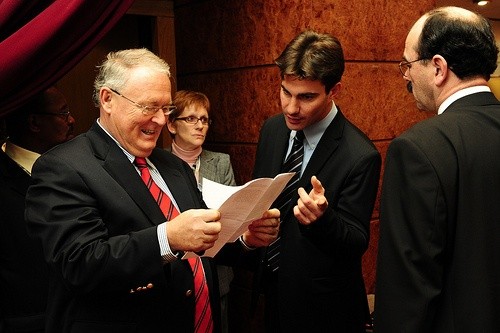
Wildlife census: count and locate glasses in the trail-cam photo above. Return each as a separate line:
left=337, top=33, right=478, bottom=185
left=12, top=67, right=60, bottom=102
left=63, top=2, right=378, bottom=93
left=398, top=57, right=454, bottom=76
left=109, top=89, right=177, bottom=115
left=34, top=109, right=72, bottom=118
left=173, top=117, right=210, bottom=125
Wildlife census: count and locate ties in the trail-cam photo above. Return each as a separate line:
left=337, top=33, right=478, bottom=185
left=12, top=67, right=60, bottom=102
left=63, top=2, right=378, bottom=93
left=136, top=156, right=213, bottom=333
left=260, top=129, right=303, bottom=266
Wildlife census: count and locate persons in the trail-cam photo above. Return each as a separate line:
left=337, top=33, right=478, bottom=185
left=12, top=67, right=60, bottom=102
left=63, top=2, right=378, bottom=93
left=159, top=91, right=237, bottom=333
left=250, top=31, right=382, bottom=333
left=25, top=49, right=281, bottom=333
left=373, top=5, right=500, bottom=333
left=0, top=84, right=79, bottom=333
